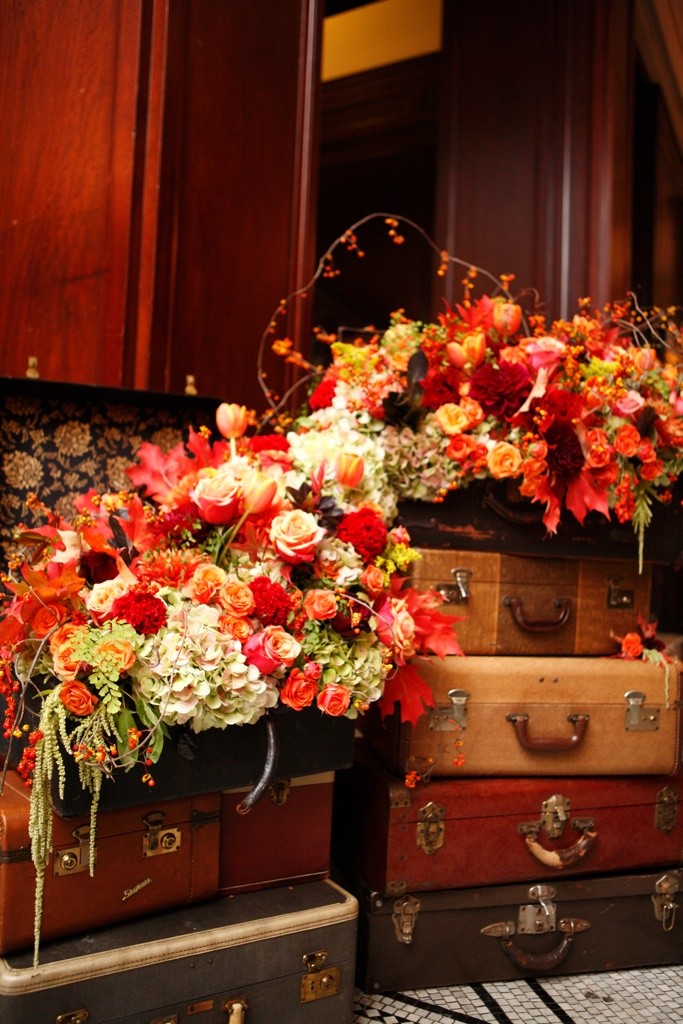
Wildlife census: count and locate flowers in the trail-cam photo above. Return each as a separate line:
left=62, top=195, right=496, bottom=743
left=0, top=213, right=683, bottom=968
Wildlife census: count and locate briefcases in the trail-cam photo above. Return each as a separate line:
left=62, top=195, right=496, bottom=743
left=0, top=476, right=683, bottom=1024
